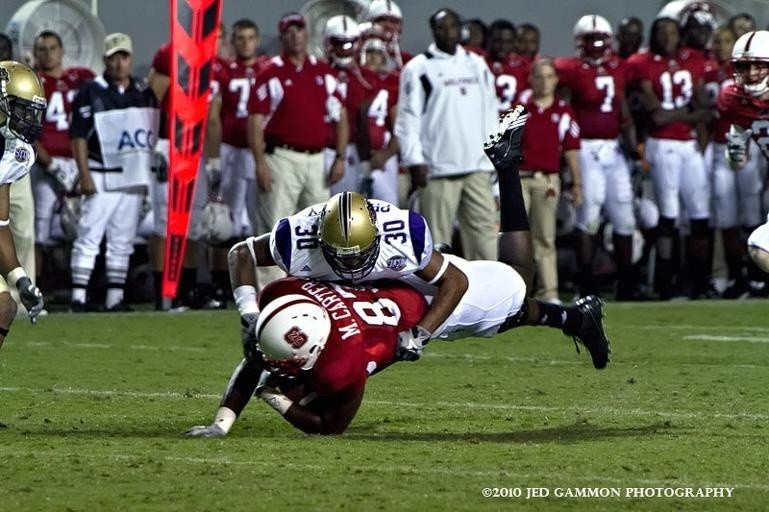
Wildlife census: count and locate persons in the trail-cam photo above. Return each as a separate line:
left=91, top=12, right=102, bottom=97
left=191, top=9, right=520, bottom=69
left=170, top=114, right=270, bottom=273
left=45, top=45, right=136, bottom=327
left=0, top=59, right=45, bottom=360
left=185, top=240, right=613, bottom=438
left=0, top=0, right=768, bottom=317
left=225, top=194, right=468, bottom=363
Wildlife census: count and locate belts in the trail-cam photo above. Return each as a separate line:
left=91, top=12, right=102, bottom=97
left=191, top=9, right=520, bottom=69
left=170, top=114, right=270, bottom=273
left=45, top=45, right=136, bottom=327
left=272, top=138, right=324, bottom=155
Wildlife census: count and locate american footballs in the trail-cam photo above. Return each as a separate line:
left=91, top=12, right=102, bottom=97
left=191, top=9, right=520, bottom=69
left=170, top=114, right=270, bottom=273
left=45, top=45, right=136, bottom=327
left=279, top=375, right=321, bottom=418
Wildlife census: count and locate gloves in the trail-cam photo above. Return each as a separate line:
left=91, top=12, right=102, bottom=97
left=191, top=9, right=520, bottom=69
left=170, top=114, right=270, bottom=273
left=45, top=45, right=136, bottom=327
left=183, top=419, right=226, bottom=438
left=15, top=276, right=44, bottom=326
left=396, top=326, right=431, bottom=362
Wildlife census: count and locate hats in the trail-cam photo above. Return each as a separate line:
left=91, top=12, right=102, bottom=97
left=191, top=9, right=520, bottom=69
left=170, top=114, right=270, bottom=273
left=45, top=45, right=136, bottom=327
left=279, top=13, right=305, bottom=34
left=102, top=32, right=133, bottom=59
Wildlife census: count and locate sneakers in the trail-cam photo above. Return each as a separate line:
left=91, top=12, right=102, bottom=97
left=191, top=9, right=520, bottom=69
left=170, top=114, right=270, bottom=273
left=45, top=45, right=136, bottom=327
left=39, top=274, right=234, bottom=311
left=562, top=296, right=611, bottom=372
left=558, top=268, right=765, bottom=300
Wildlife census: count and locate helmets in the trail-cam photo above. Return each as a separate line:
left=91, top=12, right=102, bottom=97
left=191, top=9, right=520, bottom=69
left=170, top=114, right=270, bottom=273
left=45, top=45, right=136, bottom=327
left=323, top=2, right=404, bottom=74
left=251, top=293, right=331, bottom=380
left=315, top=187, right=383, bottom=282
left=729, top=29, right=768, bottom=100
left=0, top=61, right=48, bottom=145
left=573, top=13, right=612, bottom=65
